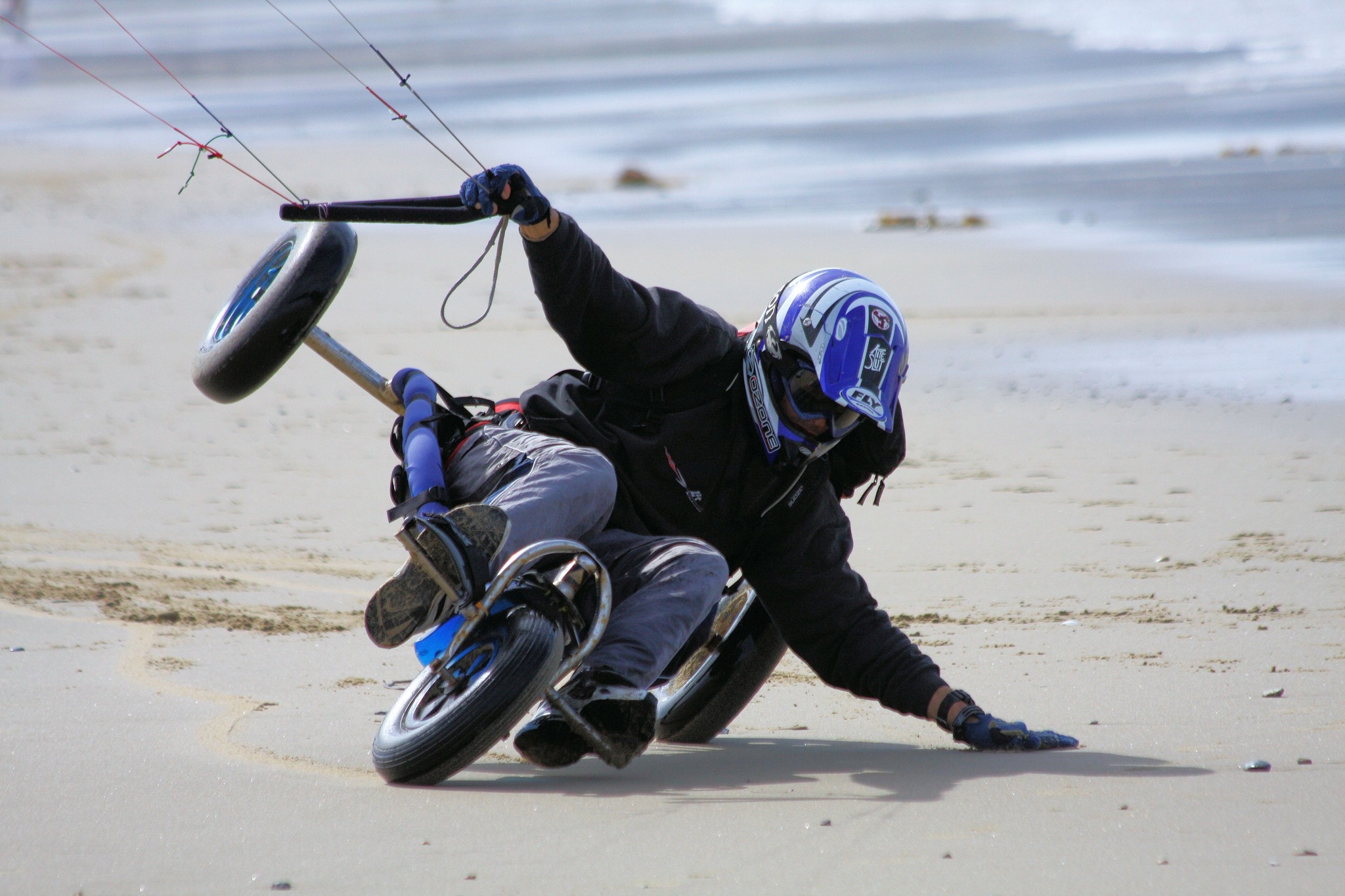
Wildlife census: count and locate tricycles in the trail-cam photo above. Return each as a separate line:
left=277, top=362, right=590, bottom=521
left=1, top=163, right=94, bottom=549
left=193, top=220, right=789, bottom=788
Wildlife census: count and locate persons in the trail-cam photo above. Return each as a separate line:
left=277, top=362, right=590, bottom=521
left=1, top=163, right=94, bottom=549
left=361, top=162, right=1078, bottom=769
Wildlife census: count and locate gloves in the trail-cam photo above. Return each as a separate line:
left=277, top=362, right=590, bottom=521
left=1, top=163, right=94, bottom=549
left=952, top=707, right=1077, bottom=751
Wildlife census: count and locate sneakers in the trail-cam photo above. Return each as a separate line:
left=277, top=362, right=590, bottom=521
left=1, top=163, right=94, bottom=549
left=512, top=666, right=660, bottom=770
left=365, top=504, right=513, bottom=649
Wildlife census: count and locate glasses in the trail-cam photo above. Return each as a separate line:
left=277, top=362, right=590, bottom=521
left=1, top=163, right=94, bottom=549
left=782, top=357, right=866, bottom=438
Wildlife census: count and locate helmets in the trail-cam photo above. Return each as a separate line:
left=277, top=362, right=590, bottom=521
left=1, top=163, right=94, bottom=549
left=743, top=267, right=910, bottom=461
